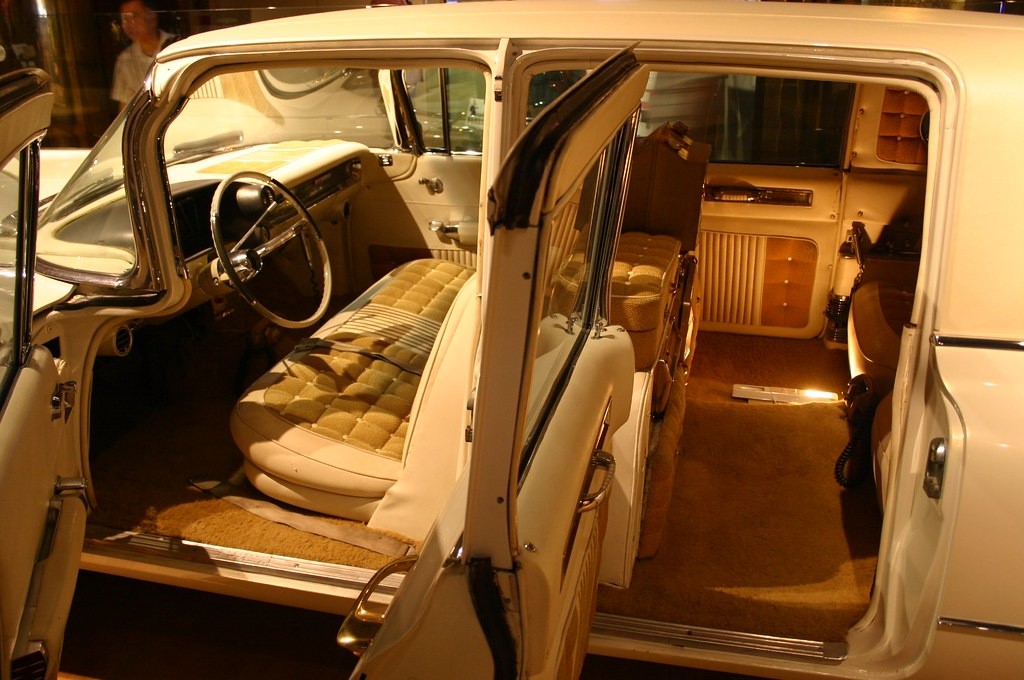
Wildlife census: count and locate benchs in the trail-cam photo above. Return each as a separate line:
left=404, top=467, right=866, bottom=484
left=230, top=258, right=476, bottom=521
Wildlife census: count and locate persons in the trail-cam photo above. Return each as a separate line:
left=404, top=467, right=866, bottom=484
left=111, top=0, right=174, bottom=114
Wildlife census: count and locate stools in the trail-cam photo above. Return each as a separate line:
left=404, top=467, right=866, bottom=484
left=548, top=231, right=682, bottom=370
left=846, top=281, right=916, bottom=395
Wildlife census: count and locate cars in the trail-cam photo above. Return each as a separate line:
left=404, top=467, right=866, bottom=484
left=0, top=0, right=1024, bottom=680
